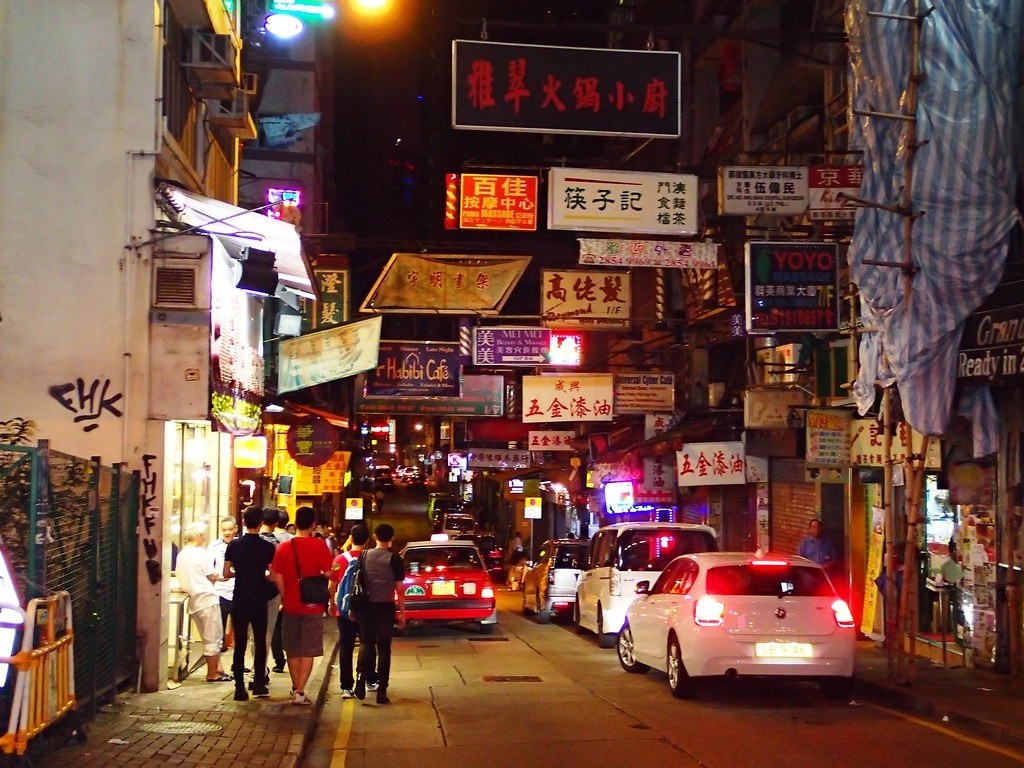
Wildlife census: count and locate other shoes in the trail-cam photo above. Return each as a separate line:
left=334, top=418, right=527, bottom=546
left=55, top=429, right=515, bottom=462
left=252, top=687, right=272, bottom=697
left=272, top=663, right=284, bottom=673
left=231, top=662, right=250, bottom=674
left=375, top=694, right=390, bottom=704
left=234, top=689, right=250, bottom=702
left=368, top=681, right=379, bottom=692
left=340, top=689, right=354, bottom=699
left=291, top=692, right=313, bottom=705
left=355, top=672, right=366, bottom=699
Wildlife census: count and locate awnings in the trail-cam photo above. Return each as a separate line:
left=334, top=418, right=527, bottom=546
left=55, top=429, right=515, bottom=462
left=155, top=180, right=324, bottom=301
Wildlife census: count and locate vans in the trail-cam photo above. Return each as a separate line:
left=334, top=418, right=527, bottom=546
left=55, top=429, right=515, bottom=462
left=572, top=520, right=721, bottom=648
left=522, top=538, right=611, bottom=625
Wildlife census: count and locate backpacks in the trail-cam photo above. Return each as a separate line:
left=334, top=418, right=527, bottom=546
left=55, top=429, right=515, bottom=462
left=336, top=552, right=359, bottom=619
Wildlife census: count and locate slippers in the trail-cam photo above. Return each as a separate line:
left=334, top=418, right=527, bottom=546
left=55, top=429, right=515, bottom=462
left=205, top=674, right=232, bottom=682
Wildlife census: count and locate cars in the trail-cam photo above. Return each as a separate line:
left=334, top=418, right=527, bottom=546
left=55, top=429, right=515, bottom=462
left=392, top=534, right=499, bottom=638
left=616, top=550, right=857, bottom=699
left=373, top=464, right=505, bottom=582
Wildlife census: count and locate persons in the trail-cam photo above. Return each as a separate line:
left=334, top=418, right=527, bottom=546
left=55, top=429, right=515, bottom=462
left=270, top=506, right=341, bottom=704
left=175, top=522, right=234, bottom=682
left=258, top=502, right=337, bottom=550
left=208, top=516, right=252, bottom=673
left=349, top=523, right=405, bottom=704
left=797, top=518, right=840, bottom=570
left=223, top=506, right=277, bottom=701
left=328, top=523, right=379, bottom=698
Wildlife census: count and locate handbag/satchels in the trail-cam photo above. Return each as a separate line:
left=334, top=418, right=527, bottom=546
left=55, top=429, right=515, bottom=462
left=238, top=569, right=281, bottom=607
left=299, top=575, right=330, bottom=604
left=351, top=568, right=371, bottom=606
